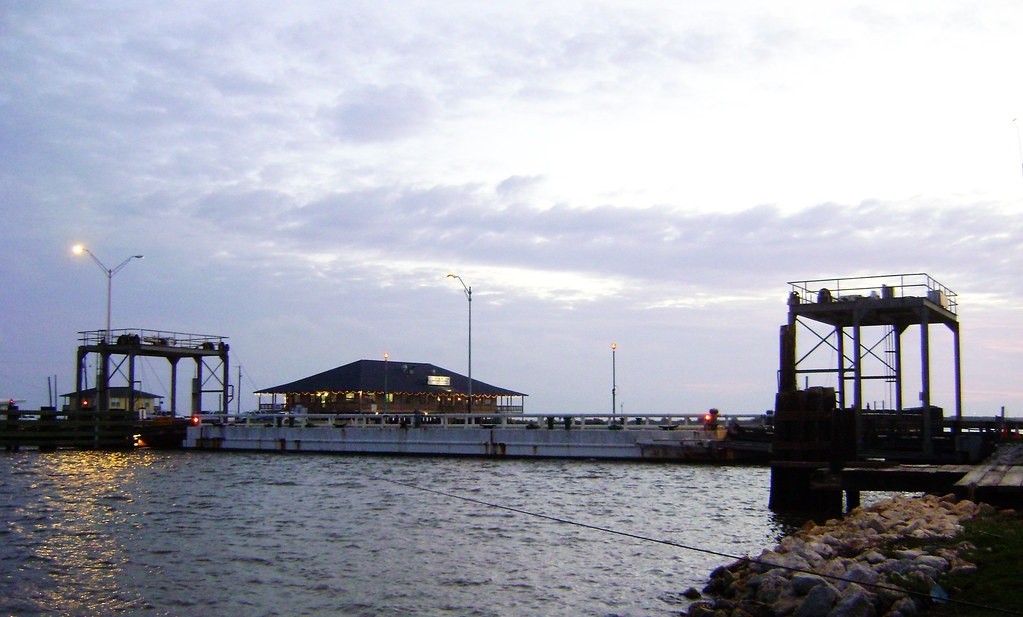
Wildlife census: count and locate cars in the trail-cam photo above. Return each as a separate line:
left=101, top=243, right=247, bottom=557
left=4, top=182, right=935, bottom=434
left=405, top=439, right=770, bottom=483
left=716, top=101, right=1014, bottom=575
left=147, top=410, right=185, bottom=420
left=235, top=410, right=267, bottom=423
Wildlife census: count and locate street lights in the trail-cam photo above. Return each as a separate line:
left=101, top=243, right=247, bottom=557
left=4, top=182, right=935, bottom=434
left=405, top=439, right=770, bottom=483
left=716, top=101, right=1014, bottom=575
left=612, top=343, right=616, bottom=424
left=447, top=274, right=472, bottom=413
left=75, top=247, right=144, bottom=344
left=384, top=353, right=388, bottom=414
